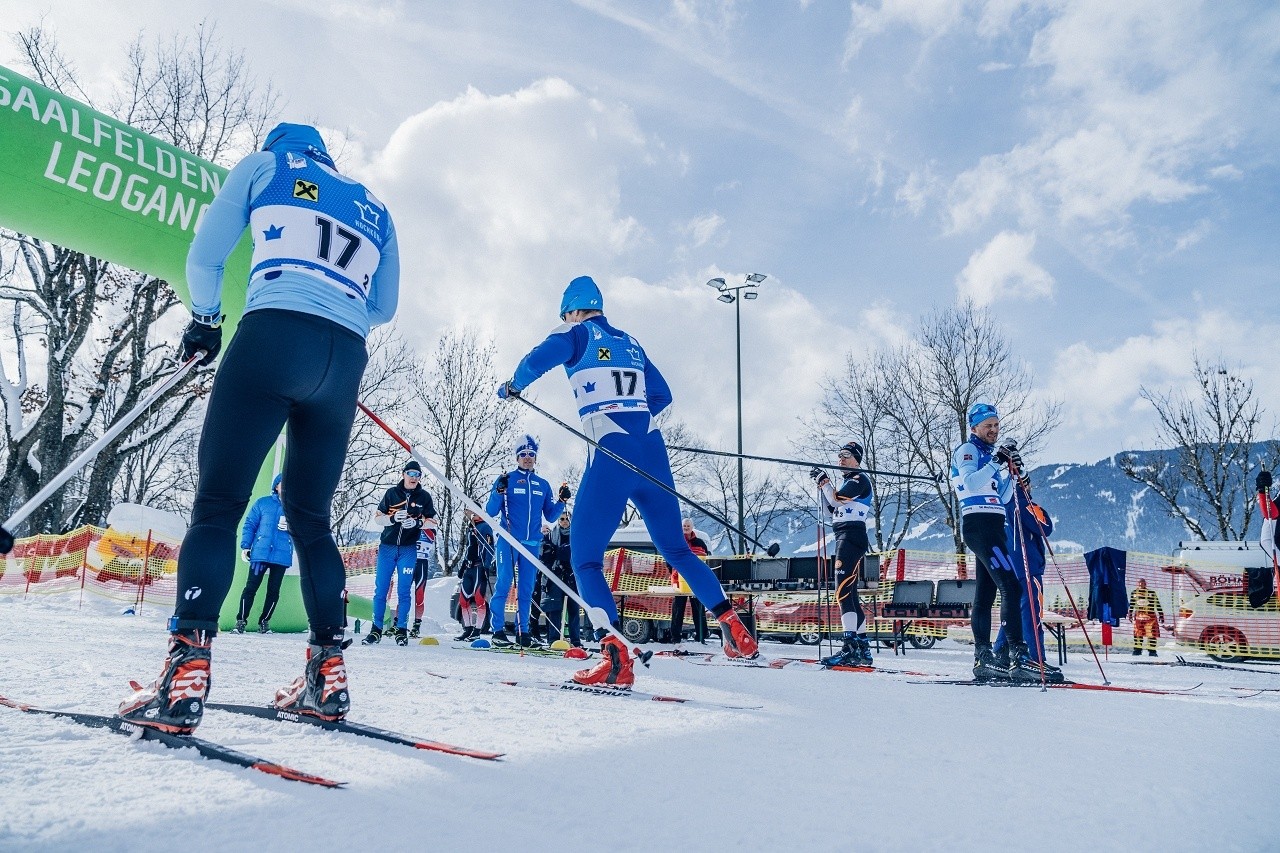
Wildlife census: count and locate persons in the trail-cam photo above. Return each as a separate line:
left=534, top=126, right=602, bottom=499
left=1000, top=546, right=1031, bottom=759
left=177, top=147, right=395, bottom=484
left=1129, top=579, right=1164, bottom=657
left=118, top=122, right=398, bottom=733
left=1256, top=471, right=1280, bottom=569
left=456, top=501, right=494, bottom=641
left=994, top=469, right=1052, bottom=663
left=385, top=529, right=436, bottom=638
left=497, top=275, right=760, bottom=689
left=529, top=506, right=580, bottom=648
left=232, top=473, right=293, bottom=634
left=665, top=518, right=710, bottom=642
left=361, top=461, right=438, bottom=646
left=485, top=434, right=571, bottom=648
left=950, top=403, right=1064, bottom=682
left=809, top=441, right=873, bottom=667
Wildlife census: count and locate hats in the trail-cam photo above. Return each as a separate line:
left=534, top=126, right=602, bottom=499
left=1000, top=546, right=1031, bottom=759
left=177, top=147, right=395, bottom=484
left=840, top=442, right=863, bottom=464
left=1015, top=469, right=1031, bottom=487
left=968, top=403, right=1000, bottom=428
left=403, top=461, right=422, bottom=475
left=561, top=276, right=603, bottom=313
left=514, top=434, right=538, bottom=457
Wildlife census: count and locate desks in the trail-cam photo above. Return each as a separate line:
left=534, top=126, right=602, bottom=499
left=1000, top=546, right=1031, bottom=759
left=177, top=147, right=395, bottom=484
left=609, top=588, right=756, bottom=643
left=750, top=588, right=885, bottom=653
left=873, top=609, right=1076, bottom=666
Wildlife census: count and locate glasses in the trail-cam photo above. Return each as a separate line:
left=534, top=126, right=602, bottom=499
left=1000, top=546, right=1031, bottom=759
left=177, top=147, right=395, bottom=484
left=403, top=471, right=421, bottom=477
left=838, top=452, right=854, bottom=458
left=559, top=517, right=571, bottom=521
left=519, top=452, right=535, bottom=458
left=559, top=295, right=579, bottom=322
left=969, top=404, right=997, bottom=419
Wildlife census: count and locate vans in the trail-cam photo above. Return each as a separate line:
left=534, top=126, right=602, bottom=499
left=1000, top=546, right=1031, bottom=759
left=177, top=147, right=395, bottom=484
left=449, top=528, right=762, bottom=658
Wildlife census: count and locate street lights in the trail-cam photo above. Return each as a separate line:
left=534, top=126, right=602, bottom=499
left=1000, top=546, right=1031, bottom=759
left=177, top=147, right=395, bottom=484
left=706, top=273, right=768, bottom=559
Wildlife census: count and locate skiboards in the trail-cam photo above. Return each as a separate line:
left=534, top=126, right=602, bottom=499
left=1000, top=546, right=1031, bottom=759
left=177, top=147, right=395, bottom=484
left=766, top=658, right=951, bottom=677
left=422, top=651, right=853, bottom=710
left=905, top=681, right=1266, bottom=698
left=0, top=681, right=508, bottom=789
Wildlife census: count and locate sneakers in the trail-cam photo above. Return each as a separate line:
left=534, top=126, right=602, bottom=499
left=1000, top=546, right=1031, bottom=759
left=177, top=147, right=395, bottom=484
left=574, top=610, right=1160, bottom=689
left=117, top=618, right=581, bottom=736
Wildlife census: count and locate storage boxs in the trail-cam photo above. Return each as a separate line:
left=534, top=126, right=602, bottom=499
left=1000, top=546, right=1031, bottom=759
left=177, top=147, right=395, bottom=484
left=926, top=578, right=976, bottom=618
left=707, top=552, right=880, bottom=589
left=881, top=580, right=936, bottom=618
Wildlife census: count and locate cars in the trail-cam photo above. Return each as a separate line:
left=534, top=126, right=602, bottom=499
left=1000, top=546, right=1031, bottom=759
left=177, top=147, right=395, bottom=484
left=758, top=590, right=940, bottom=649
left=1160, top=564, right=1280, bottom=663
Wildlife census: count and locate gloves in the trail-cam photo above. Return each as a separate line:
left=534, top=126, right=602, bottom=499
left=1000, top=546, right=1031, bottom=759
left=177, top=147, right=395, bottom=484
left=278, top=522, right=287, bottom=530
left=496, top=474, right=510, bottom=493
left=181, top=311, right=225, bottom=367
left=1007, top=451, right=1025, bottom=475
left=482, top=568, right=490, bottom=581
left=402, top=518, right=423, bottom=529
left=389, top=510, right=408, bottom=524
left=558, top=486, right=572, bottom=502
left=497, top=380, right=521, bottom=402
left=241, top=549, right=250, bottom=562
left=1255, top=470, right=1272, bottom=494
left=810, top=466, right=829, bottom=484
left=445, top=564, right=452, bottom=576
left=994, top=437, right=1018, bottom=465
